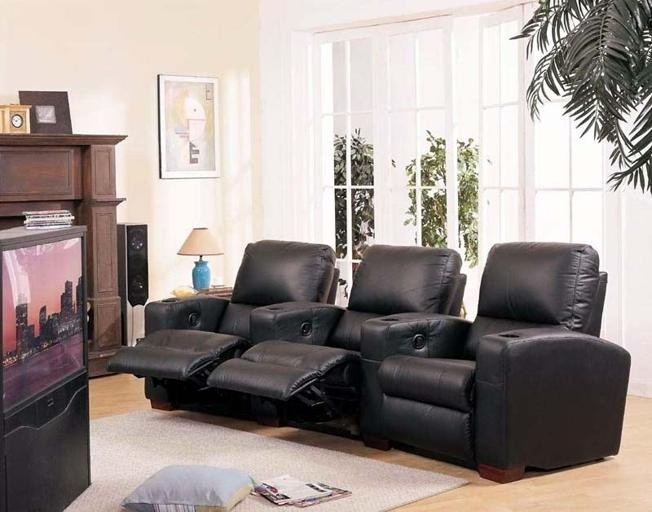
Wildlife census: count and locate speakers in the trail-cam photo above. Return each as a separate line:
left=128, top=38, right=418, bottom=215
left=117, top=222, right=151, bottom=349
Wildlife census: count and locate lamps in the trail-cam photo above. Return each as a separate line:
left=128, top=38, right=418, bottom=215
left=177, top=226, right=222, bottom=290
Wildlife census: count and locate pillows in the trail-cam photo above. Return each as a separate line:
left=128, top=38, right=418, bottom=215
left=121, top=462, right=257, bottom=512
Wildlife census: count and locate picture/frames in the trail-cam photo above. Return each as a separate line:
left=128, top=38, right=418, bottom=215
left=18, top=90, right=73, bottom=134
left=155, top=73, right=222, bottom=180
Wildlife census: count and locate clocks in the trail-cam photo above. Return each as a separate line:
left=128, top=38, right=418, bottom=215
left=0, top=106, right=29, bottom=135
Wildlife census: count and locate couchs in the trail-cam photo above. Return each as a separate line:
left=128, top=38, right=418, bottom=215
left=205, top=241, right=468, bottom=454
left=105, top=242, right=343, bottom=412
left=355, top=239, right=630, bottom=481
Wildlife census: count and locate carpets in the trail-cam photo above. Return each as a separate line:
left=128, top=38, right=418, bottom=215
left=60, top=407, right=472, bottom=512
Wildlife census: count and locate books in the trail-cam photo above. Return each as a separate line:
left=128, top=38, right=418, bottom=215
left=252, top=474, right=332, bottom=505
left=289, top=480, right=352, bottom=508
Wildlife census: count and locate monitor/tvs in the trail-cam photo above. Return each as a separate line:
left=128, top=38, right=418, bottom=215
left=0, top=225, right=88, bottom=419
